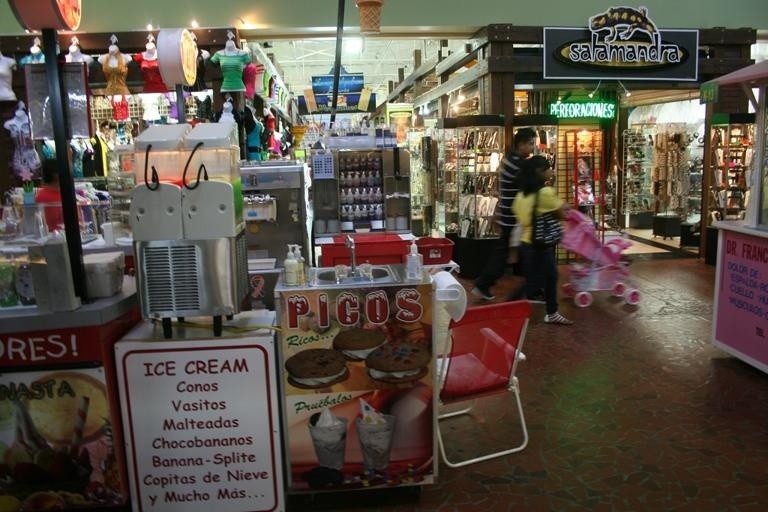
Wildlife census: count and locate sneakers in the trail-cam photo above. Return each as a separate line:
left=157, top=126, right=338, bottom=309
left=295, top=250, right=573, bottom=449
left=472, top=287, right=496, bottom=301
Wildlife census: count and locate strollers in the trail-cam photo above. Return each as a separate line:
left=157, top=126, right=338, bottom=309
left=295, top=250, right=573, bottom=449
left=562, top=208, right=641, bottom=308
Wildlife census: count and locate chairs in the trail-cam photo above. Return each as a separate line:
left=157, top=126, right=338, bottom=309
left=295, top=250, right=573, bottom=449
left=432, top=300, right=533, bottom=463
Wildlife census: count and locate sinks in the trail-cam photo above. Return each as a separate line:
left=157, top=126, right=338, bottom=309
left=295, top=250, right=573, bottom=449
left=308, top=265, right=402, bottom=287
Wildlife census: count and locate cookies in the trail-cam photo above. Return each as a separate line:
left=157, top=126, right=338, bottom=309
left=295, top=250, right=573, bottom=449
left=365, top=343, right=429, bottom=384
left=284, top=348, right=350, bottom=389
left=332, top=328, right=390, bottom=361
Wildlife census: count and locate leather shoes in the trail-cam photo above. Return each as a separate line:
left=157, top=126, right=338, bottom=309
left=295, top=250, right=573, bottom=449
left=544, top=313, right=575, bottom=326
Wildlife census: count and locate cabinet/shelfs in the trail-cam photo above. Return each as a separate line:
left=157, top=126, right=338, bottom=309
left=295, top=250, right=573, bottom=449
left=566, top=129, right=606, bottom=263
left=409, top=62, right=491, bottom=131
left=619, top=127, right=654, bottom=228
left=456, top=114, right=505, bottom=267
left=436, top=118, right=456, bottom=242
left=314, top=150, right=411, bottom=266
left=711, top=215, right=768, bottom=375
left=653, top=125, right=704, bottom=248
left=704, top=123, right=760, bottom=264
left=113, top=311, right=286, bottom=511
left=515, top=115, right=560, bottom=195
left=1, top=288, right=138, bottom=510
left=275, top=265, right=437, bottom=496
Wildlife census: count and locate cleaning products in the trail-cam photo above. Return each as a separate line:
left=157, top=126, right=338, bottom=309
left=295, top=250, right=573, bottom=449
left=361, top=204, right=369, bottom=220
left=404, top=237, right=424, bottom=285
left=374, top=171, right=381, bottom=186
left=360, top=171, right=366, bottom=186
left=341, top=206, right=348, bottom=221
left=353, top=171, right=361, bottom=186
left=346, top=159, right=352, bottom=170
left=360, top=188, right=367, bottom=203
left=352, top=159, right=359, bottom=170
left=283, top=244, right=298, bottom=285
left=339, top=158, right=346, bottom=170
left=297, top=245, right=307, bottom=286
left=368, top=188, right=374, bottom=203
left=367, top=158, right=374, bottom=170
left=354, top=188, right=361, bottom=204
left=367, top=171, right=374, bottom=186
left=360, top=158, right=367, bottom=170
left=374, top=158, right=381, bottom=170
left=375, top=187, right=380, bottom=203
left=346, top=189, right=353, bottom=204
left=375, top=205, right=382, bottom=220
left=369, top=205, right=376, bottom=220
left=339, top=172, right=346, bottom=186
left=346, top=172, right=353, bottom=187
left=348, top=205, right=354, bottom=221
left=340, top=189, right=346, bottom=205
left=355, top=205, right=361, bottom=220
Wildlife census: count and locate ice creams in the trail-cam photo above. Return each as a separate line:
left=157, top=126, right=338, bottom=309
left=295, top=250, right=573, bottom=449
left=355, top=397, right=395, bottom=472
left=306, top=406, right=348, bottom=470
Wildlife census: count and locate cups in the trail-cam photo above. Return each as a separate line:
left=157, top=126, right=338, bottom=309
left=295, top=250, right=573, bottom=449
left=356, top=414, right=396, bottom=470
left=309, top=417, right=347, bottom=472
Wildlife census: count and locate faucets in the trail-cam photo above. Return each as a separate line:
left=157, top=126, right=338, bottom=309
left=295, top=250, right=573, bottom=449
left=346, top=235, right=357, bottom=276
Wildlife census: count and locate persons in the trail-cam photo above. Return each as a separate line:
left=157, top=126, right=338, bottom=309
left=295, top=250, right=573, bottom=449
left=35, top=159, right=63, bottom=231
left=125, top=124, right=133, bottom=145
left=106, top=95, right=132, bottom=120
left=98, top=45, right=132, bottom=96
left=82, top=136, right=97, bottom=179
left=182, top=34, right=209, bottom=92
left=251, top=46, right=264, bottom=92
left=281, top=138, right=291, bottom=160
left=210, top=40, right=250, bottom=92
left=191, top=90, right=213, bottom=122
left=3, top=111, right=41, bottom=182
left=92, top=132, right=108, bottom=177
left=138, top=93, right=161, bottom=122
left=273, top=130, right=283, bottom=159
left=471, top=128, right=537, bottom=301
left=65, top=44, right=93, bottom=75
left=165, top=92, right=190, bottom=123
left=107, top=121, right=121, bottom=147
left=502, top=154, right=574, bottom=326
left=269, top=74, right=277, bottom=99
left=263, top=103, right=276, bottom=151
left=100, top=121, right=109, bottom=143
left=244, top=105, right=262, bottom=161
left=0, top=53, right=17, bottom=100
left=19, top=44, right=45, bottom=70
left=133, top=42, right=168, bottom=94
left=313, top=133, right=325, bottom=149
left=68, top=136, right=86, bottom=180
left=263, top=60, right=270, bottom=99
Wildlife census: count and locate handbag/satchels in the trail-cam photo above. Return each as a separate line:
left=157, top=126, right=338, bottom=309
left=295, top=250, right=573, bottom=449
left=532, top=189, right=562, bottom=248
